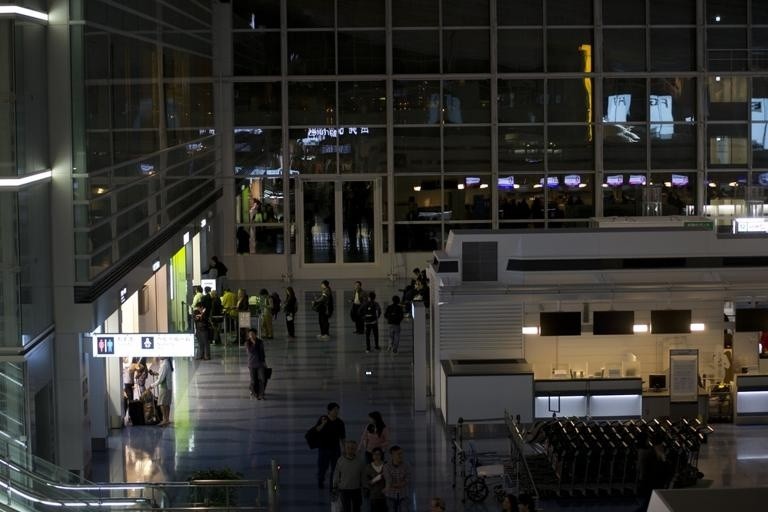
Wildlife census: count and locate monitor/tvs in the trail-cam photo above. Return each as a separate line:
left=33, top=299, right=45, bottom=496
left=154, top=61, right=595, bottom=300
left=464, top=173, right=689, bottom=190
left=540, top=312, right=581, bottom=336
left=649, top=375, right=666, bottom=388
left=736, top=309, right=768, bottom=332
left=651, top=310, right=691, bottom=334
left=593, top=311, right=635, bottom=335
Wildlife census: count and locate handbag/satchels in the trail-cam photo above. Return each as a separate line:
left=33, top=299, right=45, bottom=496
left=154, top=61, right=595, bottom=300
left=141, top=390, right=160, bottom=424
left=331, top=497, right=343, bottom=512
left=312, top=301, right=322, bottom=312
left=363, top=439, right=368, bottom=448
left=306, top=427, right=325, bottom=449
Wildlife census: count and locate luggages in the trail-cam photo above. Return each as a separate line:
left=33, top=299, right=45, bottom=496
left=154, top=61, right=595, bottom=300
left=153, top=397, right=163, bottom=421
left=129, top=400, right=145, bottom=425
left=254, top=368, right=272, bottom=394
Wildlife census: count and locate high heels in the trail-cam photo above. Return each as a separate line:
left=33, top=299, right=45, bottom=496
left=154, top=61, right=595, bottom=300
left=158, top=421, right=170, bottom=428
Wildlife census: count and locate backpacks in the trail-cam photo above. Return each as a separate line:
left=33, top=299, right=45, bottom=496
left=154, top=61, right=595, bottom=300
left=268, top=292, right=281, bottom=315
left=364, top=302, right=376, bottom=319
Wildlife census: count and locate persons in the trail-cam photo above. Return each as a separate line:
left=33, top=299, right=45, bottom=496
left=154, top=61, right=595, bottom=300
left=707, top=186, right=736, bottom=204
left=663, top=187, right=684, bottom=215
left=244, top=328, right=265, bottom=401
left=188, top=267, right=429, bottom=360
left=208, top=256, right=228, bottom=296
left=129, top=357, right=174, bottom=427
left=239, top=179, right=374, bottom=255
left=304, top=401, right=537, bottom=512
left=501, top=191, right=584, bottom=230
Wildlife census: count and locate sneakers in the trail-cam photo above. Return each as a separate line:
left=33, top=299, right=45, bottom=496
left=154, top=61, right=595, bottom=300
left=317, top=334, right=330, bottom=338
left=318, top=480, right=324, bottom=488
left=250, top=395, right=265, bottom=400
left=366, top=346, right=398, bottom=354
left=353, top=331, right=364, bottom=335
